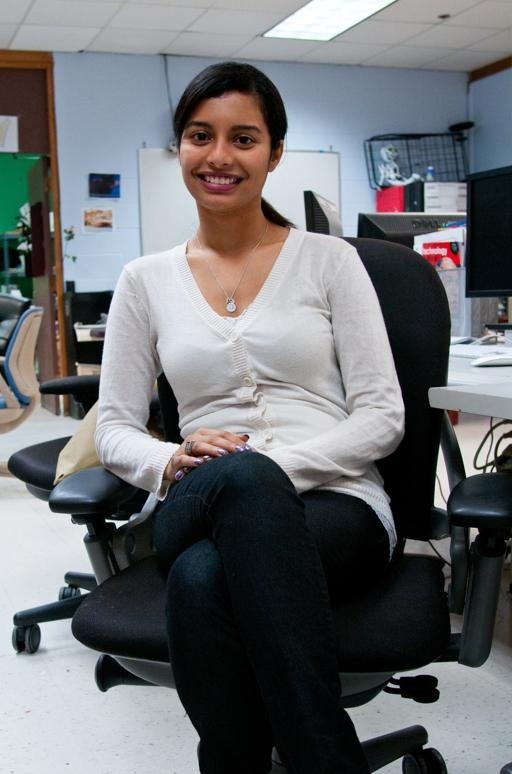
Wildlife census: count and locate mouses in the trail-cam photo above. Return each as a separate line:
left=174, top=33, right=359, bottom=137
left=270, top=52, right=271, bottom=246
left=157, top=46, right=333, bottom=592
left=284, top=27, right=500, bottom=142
left=471, top=354, right=512, bottom=367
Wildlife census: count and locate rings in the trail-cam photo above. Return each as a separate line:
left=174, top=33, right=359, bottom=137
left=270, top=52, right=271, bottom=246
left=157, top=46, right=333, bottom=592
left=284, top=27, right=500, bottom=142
left=183, top=440, right=196, bottom=455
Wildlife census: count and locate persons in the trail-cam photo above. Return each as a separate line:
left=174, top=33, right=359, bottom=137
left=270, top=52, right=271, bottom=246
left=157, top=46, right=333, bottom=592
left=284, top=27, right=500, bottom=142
left=93, top=60, right=408, bottom=774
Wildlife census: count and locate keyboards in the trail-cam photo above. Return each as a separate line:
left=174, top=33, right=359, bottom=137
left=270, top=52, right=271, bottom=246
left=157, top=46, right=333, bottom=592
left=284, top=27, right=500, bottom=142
left=449, top=343, right=506, bottom=359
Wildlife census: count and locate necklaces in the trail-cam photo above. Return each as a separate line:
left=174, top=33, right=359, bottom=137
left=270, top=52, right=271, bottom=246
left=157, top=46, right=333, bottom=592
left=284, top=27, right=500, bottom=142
left=190, top=223, right=272, bottom=313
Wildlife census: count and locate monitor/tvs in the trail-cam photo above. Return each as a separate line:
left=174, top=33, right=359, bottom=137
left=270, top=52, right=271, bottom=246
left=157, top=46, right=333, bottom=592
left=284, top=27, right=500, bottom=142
left=356, top=211, right=467, bottom=249
left=303, top=190, right=344, bottom=237
left=464, top=164, right=512, bottom=330
